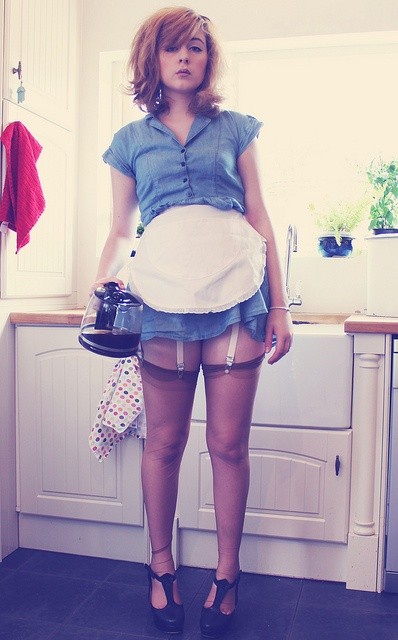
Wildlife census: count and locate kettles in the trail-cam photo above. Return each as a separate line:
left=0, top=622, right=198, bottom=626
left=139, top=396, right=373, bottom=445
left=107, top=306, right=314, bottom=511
left=76, top=282, right=144, bottom=358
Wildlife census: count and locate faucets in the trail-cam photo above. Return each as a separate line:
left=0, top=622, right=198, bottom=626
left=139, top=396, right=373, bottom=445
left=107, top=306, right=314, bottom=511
left=283, top=222, right=304, bottom=310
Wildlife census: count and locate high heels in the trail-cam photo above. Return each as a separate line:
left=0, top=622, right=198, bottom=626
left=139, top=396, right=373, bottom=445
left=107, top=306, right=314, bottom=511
left=200, top=568, right=241, bottom=638
left=144, top=564, right=184, bottom=636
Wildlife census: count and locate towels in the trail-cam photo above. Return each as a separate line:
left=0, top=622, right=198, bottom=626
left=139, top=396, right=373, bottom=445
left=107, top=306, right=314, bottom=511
left=0, top=121, right=46, bottom=254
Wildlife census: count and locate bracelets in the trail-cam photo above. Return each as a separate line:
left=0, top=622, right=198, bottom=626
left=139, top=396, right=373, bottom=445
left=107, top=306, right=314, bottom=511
left=269, top=306, right=291, bottom=310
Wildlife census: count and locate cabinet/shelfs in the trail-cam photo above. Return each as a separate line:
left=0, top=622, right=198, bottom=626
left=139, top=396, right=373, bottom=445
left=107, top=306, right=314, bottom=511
left=174, top=325, right=354, bottom=585
left=5, top=312, right=153, bottom=566
left=0, top=1, right=77, bottom=301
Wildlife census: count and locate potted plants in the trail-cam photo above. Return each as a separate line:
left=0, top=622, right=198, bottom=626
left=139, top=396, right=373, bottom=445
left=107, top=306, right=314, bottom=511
left=307, top=199, right=366, bottom=258
left=367, top=157, right=398, bottom=234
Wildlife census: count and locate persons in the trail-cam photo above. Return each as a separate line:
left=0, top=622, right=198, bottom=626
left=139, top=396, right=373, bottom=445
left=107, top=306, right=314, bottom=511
left=80, top=6, right=294, bottom=638
